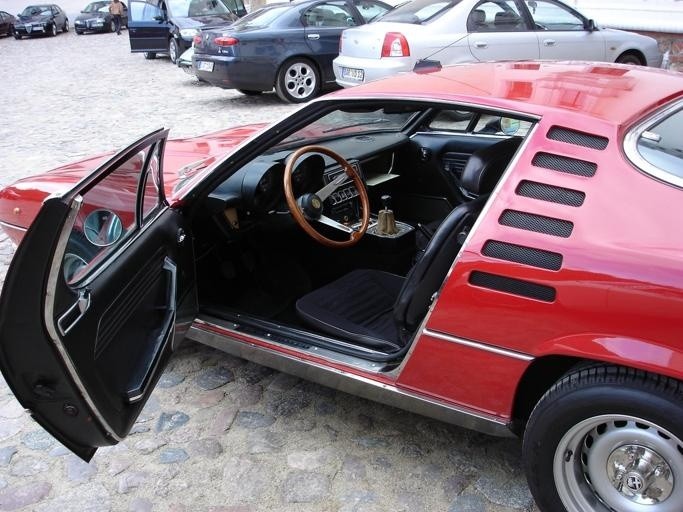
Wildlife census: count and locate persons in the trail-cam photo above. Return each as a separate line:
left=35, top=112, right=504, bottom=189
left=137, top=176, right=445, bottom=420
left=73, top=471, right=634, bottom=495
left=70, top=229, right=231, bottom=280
left=109, top=0, right=124, bottom=35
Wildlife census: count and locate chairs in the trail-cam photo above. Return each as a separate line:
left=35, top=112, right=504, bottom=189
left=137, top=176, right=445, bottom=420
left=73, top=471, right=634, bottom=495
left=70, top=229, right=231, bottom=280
left=495, top=12, right=519, bottom=29
left=472, top=10, right=488, bottom=29
left=292, top=134, right=522, bottom=355
